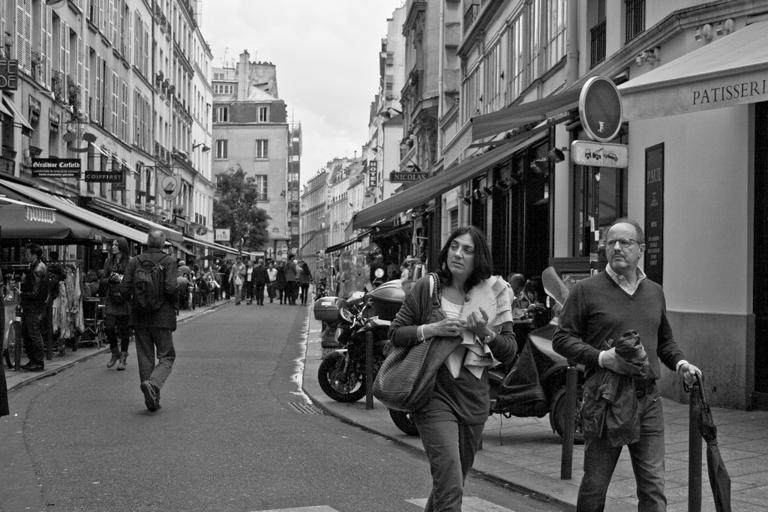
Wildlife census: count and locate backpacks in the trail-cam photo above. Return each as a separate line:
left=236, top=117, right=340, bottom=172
left=132, top=256, right=168, bottom=317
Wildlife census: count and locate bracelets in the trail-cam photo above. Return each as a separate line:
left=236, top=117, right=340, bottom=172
left=420, top=322, right=427, bottom=343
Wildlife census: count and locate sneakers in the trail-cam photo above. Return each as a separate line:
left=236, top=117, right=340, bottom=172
left=21, top=360, right=45, bottom=370
left=140, top=380, right=159, bottom=412
left=235, top=297, right=307, bottom=306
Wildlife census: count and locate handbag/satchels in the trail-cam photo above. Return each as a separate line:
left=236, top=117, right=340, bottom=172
left=371, top=304, right=461, bottom=413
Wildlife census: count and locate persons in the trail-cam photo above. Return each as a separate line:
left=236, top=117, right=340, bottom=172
left=552, top=216, right=702, bottom=511
left=177, top=255, right=311, bottom=308
left=82, top=269, right=105, bottom=297
left=387, top=255, right=400, bottom=278
left=0, top=245, right=64, bottom=419
left=389, top=224, right=519, bottom=511
left=370, top=256, right=384, bottom=281
left=100, top=238, right=135, bottom=370
left=120, top=230, right=180, bottom=412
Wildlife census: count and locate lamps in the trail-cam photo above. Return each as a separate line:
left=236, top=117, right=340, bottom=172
left=457, top=146, right=571, bottom=208
left=715, top=18, right=736, bottom=37
left=694, top=24, right=714, bottom=44
left=369, top=143, right=384, bottom=152
left=192, top=142, right=211, bottom=154
left=634, top=56, right=658, bottom=69
left=640, top=47, right=661, bottom=62
left=378, top=104, right=404, bottom=123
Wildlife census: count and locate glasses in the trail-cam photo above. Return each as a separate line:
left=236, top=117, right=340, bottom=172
left=604, top=238, right=640, bottom=247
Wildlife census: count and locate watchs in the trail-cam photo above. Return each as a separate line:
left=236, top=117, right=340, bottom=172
left=483, top=333, right=494, bottom=343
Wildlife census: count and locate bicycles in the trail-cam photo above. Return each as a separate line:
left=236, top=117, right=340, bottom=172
left=5, top=287, right=32, bottom=368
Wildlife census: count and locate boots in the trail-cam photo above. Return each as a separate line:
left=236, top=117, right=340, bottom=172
left=106, top=346, right=120, bottom=367
left=117, top=351, right=129, bottom=370
